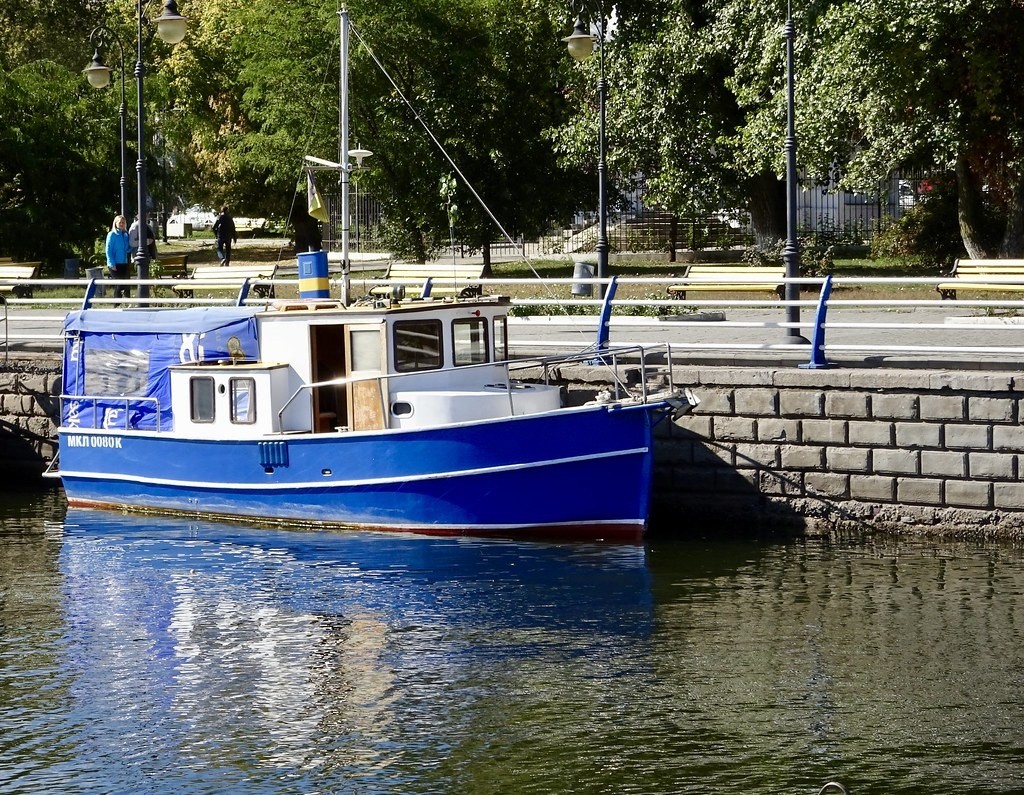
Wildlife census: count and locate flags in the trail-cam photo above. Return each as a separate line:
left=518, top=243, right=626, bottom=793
left=305, top=162, right=330, bottom=224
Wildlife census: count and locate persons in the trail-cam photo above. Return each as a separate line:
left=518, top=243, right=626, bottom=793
left=106, top=214, right=159, bottom=308
left=212, top=206, right=237, bottom=267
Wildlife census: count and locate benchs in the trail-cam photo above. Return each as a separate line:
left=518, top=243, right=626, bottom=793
left=936, top=259, right=1024, bottom=308
left=0, top=256, right=43, bottom=299
left=212, top=217, right=267, bottom=238
left=368, top=263, right=487, bottom=299
left=172, top=264, right=279, bottom=299
left=103, top=255, right=188, bottom=289
left=319, top=412, right=337, bottom=432
left=666, top=265, right=788, bottom=300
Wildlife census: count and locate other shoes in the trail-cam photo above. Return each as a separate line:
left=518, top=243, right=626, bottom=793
left=225, top=261, right=229, bottom=268
left=220, top=259, right=227, bottom=267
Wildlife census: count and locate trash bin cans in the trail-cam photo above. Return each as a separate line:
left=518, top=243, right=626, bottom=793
left=85, top=266, right=107, bottom=298
left=571, top=262, right=595, bottom=298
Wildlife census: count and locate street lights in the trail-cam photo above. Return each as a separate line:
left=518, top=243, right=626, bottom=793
left=561, top=0, right=610, bottom=300
left=80, top=26, right=127, bottom=228
left=133, top=0, right=190, bottom=308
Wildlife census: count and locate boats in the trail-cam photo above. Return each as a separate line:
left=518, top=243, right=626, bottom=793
left=40, top=2, right=700, bottom=543
